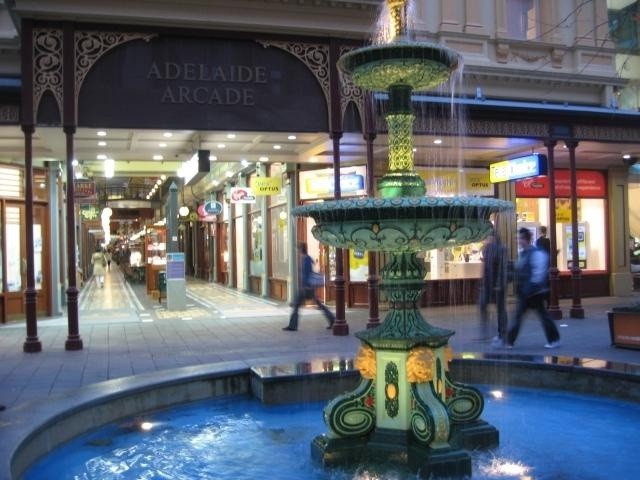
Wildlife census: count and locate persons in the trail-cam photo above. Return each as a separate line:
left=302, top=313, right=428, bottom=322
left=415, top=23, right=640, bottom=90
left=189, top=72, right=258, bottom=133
left=500, top=226, right=561, bottom=351
left=472, top=231, right=509, bottom=342
left=105, top=249, right=113, bottom=272
left=91, top=248, right=108, bottom=289
left=534, top=226, right=560, bottom=308
left=281, top=241, right=336, bottom=331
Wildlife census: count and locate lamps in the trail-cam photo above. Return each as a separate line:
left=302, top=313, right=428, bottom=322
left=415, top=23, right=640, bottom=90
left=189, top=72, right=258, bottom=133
left=179, top=182, right=189, bottom=216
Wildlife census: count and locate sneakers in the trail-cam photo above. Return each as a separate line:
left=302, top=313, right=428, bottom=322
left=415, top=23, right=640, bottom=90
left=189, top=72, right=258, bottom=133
left=282, top=326, right=297, bottom=331
left=491, top=336, right=562, bottom=349
left=326, top=317, right=336, bottom=330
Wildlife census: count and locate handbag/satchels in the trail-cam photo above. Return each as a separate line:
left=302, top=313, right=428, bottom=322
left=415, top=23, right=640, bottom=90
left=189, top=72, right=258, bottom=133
left=305, top=271, right=325, bottom=287
